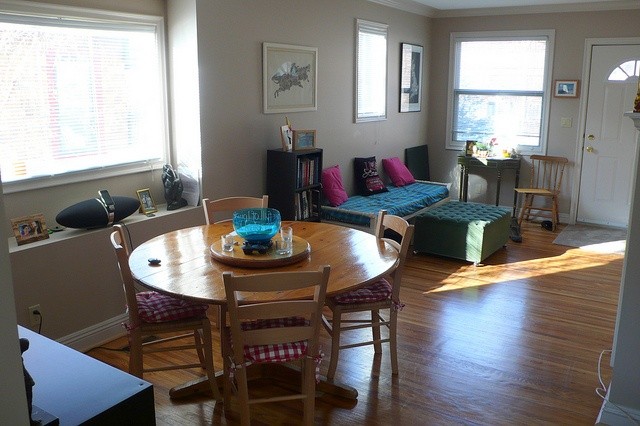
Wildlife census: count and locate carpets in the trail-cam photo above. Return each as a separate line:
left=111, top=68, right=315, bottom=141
left=551, top=223, right=626, bottom=255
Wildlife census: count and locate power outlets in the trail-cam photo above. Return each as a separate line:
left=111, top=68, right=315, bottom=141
left=28, top=302, right=45, bottom=327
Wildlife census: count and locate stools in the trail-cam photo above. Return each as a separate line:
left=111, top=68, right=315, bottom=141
left=409, top=198, right=511, bottom=268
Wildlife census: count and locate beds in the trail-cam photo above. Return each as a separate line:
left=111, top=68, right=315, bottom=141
left=317, top=179, right=453, bottom=235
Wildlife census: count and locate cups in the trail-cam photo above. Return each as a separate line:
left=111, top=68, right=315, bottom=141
left=280, top=226, right=292, bottom=242
left=275, top=238, right=292, bottom=257
left=221, top=234, right=234, bottom=251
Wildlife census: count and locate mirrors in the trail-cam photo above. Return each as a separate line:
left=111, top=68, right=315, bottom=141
left=347, top=16, right=394, bottom=126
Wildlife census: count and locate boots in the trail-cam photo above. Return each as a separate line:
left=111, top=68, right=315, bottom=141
left=509, top=217, right=522, bottom=242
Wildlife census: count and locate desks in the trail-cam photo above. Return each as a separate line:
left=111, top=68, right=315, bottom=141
left=455, top=152, right=523, bottom=219
left=127, top=219, right=400, bottom=403
left=18, top=326, right=158, bottom=426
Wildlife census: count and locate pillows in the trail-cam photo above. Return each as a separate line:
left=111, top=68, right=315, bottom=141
left=383, top=155, right=416, bottom=187
left=354, top=155, right=389, bottom=196
left=322, top=163, right=348, bottom=206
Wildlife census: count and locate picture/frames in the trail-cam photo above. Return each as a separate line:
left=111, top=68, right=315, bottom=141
left=134, top=186, right=158, bottom=216
left=10, top=211, right=50, bottom=247
left=258, top=40, right=322, bottom=115
left=278, top=125, right=291, bottom=152
left=396, top=40, right=424, bottom=114
left=550, top=78, right=580, bottom=101
left=292, top=129, right=318, bottom=152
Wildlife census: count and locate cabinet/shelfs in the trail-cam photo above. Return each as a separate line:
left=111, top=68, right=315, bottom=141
left=265, top=148, right=324, bottom=223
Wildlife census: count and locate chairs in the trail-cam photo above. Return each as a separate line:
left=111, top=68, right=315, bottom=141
left=108, top=222, right=222, bottom=403
left=199, top=194, right=270, bottom=229
left=321, top=209, right=416, bottom=383
left=221, top=262, right=333, bottom=425
left=511, top=153, right=571, bottom=233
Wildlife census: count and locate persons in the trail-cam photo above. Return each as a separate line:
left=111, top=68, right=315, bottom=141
left=141, top=194, right=153, bottom=207
left=20, top=221, right=40, bottom=237
